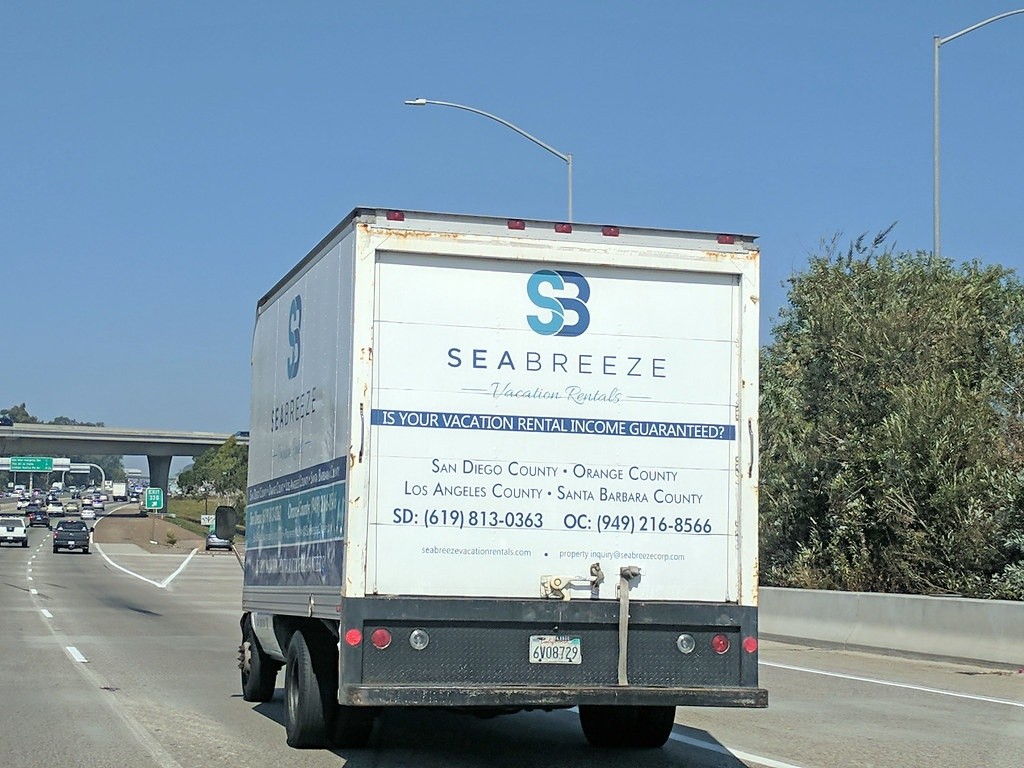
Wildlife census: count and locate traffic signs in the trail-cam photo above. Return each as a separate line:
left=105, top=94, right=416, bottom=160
left=146, top=486, right=165, bottom=511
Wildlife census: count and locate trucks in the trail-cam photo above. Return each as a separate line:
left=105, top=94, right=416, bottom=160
left=215, top=204, right=868, bottom=750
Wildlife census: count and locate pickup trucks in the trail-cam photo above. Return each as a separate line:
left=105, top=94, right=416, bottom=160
left=48, top=518, right=94, bottom=553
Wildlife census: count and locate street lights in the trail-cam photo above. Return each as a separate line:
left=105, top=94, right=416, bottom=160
left=402, top=96, right=574, bottom=223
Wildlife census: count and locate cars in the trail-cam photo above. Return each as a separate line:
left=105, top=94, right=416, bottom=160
left=0, top=481, right=144, bottom=521
left=30, top=511, right=49, bottom=527
left=204, top=529, right=232, bottom=552
left=0, top=515, right=29, bottom=548
